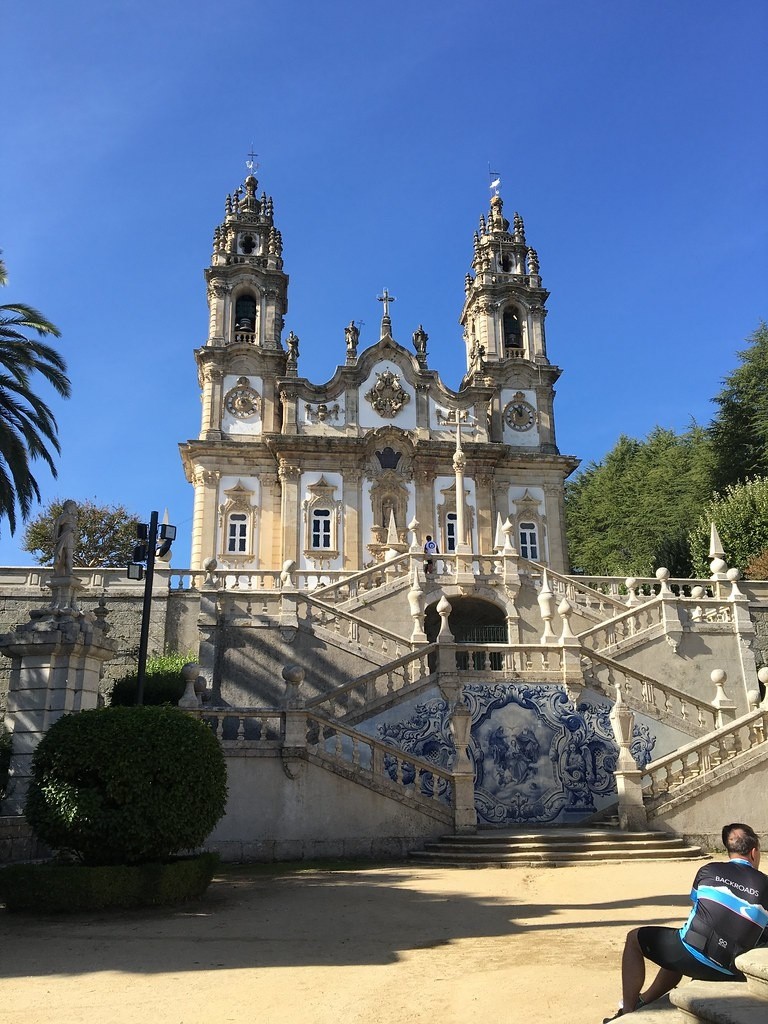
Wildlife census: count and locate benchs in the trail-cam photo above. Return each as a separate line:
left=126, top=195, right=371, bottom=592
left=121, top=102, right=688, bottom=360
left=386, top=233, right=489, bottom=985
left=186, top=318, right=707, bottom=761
left=668, top=978, right=768, bottom=1024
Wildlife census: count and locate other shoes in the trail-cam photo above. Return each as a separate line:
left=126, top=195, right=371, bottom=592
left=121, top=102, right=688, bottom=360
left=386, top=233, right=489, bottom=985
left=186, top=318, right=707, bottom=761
left=634, top=993, right=645, bottom=1009
left=603, top=1009, right=624, bottom=1024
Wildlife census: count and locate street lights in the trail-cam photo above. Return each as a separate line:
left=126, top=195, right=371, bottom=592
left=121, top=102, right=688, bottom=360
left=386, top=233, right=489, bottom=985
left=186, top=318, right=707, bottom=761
left=127, top=511, right=176, bottom=706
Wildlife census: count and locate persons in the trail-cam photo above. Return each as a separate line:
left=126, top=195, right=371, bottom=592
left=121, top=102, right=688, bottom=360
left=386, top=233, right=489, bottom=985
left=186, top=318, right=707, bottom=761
left=423, top=535, right=440, bottom=573
left=285, top=330, right=300, bottom=362
left=412, top=324, right=430, bottom=352
left=470, top=341, right=486, bottom=371
left=344, top=319, right=360, bottom=352
left=602, top=822, right=768, bottom=1024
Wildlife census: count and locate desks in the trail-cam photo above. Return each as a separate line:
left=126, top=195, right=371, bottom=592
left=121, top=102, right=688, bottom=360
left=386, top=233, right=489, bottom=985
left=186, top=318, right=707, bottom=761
left=734, top=943, right=768, bottom=998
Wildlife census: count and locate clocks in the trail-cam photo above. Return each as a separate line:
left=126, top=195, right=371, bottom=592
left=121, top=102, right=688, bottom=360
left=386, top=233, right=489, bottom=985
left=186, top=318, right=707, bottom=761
left=505, top=401, right=536, bottom=432
left=224, top=386, right=261, bottom=419
